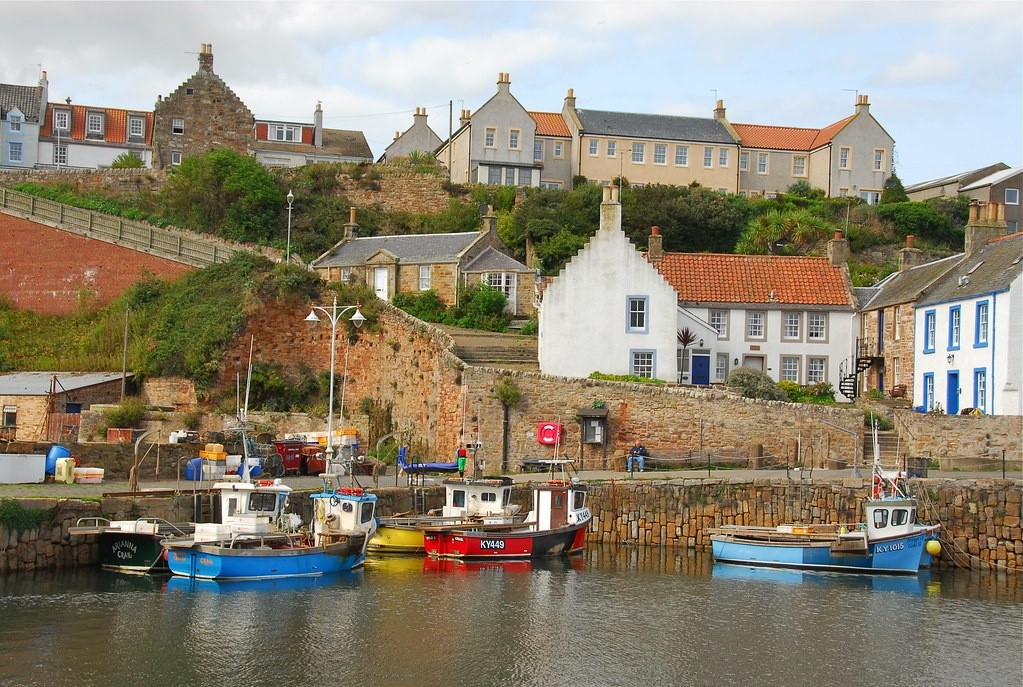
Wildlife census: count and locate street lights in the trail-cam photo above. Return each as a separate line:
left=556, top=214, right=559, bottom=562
left=303, top=293, right=367, bottom=479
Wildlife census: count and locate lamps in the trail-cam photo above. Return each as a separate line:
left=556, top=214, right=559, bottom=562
left=735, top=358, right=738, bottom=365
left=700, top=339, right=703, bottom=347
left=947, top=354, right=954, bottom=364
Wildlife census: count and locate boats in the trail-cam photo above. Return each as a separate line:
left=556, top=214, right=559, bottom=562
left=709, top=408, right=941, bottom=574
left=157, top=425, right=381, bottom=580
left=710, top=559, right=942, bottom=597
left=418, top=418, right=593, bottom=560
left=398, top=445, right=460, bottom=474
left=65, top=514, right=201, bottom=578
left=365, top=440, right=519, bottom=554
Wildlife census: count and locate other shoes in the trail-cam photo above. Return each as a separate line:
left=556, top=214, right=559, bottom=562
left=627, top=470, right=631, bottom=472
left=640, top=470, right=643, bottom=472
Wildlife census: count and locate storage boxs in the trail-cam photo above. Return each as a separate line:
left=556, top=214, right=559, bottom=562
left=318, top=428, right=356, bottom=445
left=110, top=520, right=159, bottom=534
left=185, top=443, right=261, bottom=481
left=484, top=519, right=513, bottom=524
left=194, top=516, right=269, bottom=542
left=55, top=457, right=104, bottom=485
left=777, top=526, right=815, bottom=534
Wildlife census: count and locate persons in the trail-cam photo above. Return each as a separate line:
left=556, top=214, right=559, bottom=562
left=627, top=440, right=647, bottom=471
left=457, top=442, right=466, bottom=477
left=398, top=444, right=409, bottom=477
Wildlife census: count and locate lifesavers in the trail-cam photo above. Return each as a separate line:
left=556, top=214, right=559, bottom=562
left=548, top=480, right=570, bottom=487
left=253, top=479, right=274, bottom=487
left=337, top=487, right=363, bottom=497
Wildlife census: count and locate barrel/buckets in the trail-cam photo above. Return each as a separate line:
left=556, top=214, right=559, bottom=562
left=906, top=457, right=927, bottom=479
left=170, top=436, right=177, bottom=443
left=185, top=443, right=261, bottom=480
left=55, top=458, right=76, bottom=484
left=45, top=445, right=72, bottom=475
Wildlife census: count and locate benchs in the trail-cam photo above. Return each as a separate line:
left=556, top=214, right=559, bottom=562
left=637, top=448, right=657, bottom=471
left=889, top=384, right=907, bottom=399
left=907, top=457, right=927, bottom=478
